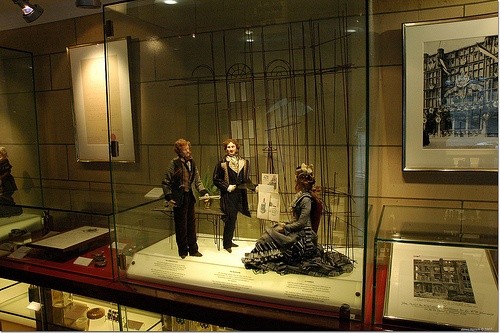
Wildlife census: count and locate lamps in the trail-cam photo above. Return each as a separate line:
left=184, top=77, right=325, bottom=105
left=12, top=0, right=44, bottom=23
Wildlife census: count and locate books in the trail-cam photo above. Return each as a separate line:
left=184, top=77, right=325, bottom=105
left=199, top=195, right=221, bottom=200
left=256, top=174, right=280, bottom=223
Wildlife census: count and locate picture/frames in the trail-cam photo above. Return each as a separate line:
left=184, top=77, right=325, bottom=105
left=382, top=236, right=496, bottom=331
left=64, top=36, right=141, bottom=165
left=400, top=12, right=498, bottom=173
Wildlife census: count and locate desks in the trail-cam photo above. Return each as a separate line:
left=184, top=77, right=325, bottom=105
left=151, top=206, right=227, bottom=250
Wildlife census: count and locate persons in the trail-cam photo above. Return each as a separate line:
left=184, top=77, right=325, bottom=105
left=213, top=139, right=258, bottom=253
left=162, top=139, right=211, bottom=258
left=245, top=163, right=323, bottom=269
left=0, top=147, right=18, bottom=195
